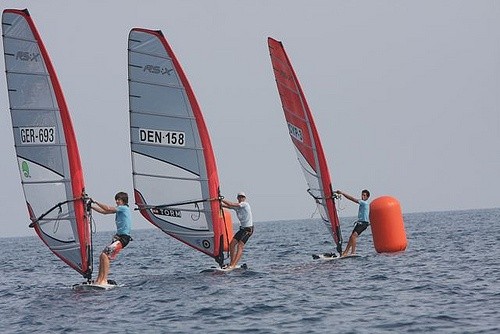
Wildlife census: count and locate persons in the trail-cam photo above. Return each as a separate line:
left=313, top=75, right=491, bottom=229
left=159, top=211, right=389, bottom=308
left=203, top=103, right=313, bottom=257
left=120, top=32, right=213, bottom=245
left=221, top=191, right=255, bottom=271
left=88, top=192, right=132, bottom=287
left=334, top=188, right=371, bottom=257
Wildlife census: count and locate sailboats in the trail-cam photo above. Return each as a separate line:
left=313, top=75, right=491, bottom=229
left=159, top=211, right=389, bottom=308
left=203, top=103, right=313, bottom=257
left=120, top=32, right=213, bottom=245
left=1, top=8, right=125, bottom=292
left=266, top=36, right=360, bottom=260
left=128, top=27, right=246, bottom=274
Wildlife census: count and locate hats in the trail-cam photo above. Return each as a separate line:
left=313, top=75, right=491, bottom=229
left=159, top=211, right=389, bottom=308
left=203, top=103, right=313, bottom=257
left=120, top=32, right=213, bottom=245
left=237, top=192, right=247, bottom=199
